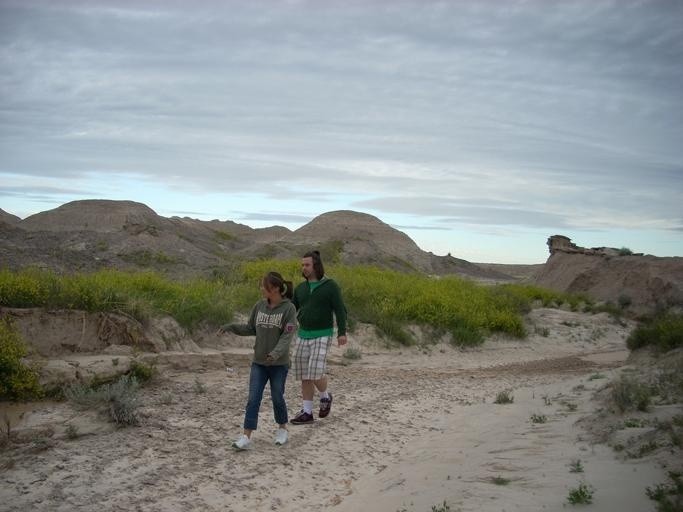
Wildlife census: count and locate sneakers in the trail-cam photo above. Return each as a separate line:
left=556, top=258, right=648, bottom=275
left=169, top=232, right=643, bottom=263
left=231, top=433, right=254, bottom=450
left=318, top=393, right=333, bottom=418
left=290, top=409, right=314, bottom=425
left=274, top=427, right=290, bottom=446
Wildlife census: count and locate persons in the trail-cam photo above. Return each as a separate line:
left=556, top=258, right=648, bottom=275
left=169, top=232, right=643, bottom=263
left=290, top=249, right=347, bottom=426
left=215, top=271, right=299, bottom=452
